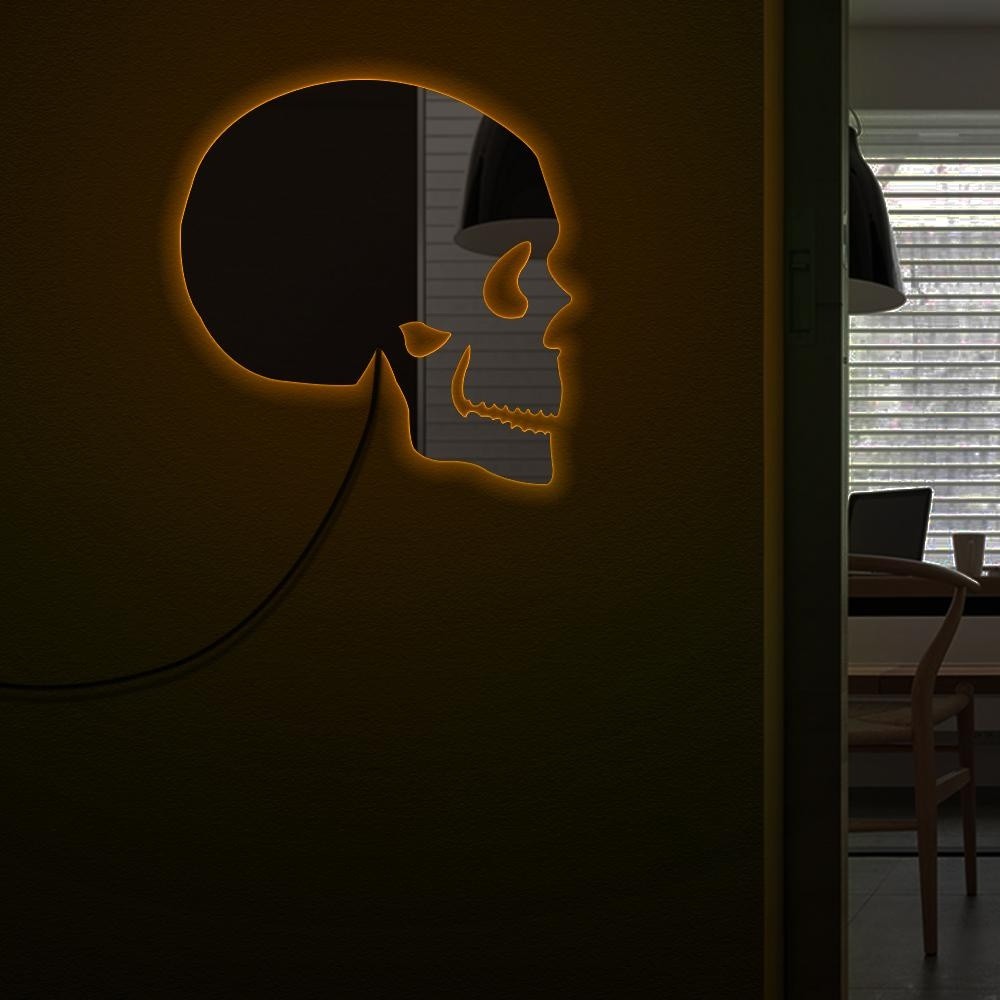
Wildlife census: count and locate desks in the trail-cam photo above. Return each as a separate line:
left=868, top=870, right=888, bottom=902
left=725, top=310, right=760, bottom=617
left=847, top=570, right=1000, bottom=791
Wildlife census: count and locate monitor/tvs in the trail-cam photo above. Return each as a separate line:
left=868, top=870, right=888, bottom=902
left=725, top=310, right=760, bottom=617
left=846, top=487, right=934, bottom=561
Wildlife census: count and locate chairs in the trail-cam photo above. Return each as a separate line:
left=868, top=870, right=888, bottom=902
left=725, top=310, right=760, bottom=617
left=848, top=552, right=983, bottom=955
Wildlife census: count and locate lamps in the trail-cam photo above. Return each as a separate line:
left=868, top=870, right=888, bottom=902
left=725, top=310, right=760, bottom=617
left=455, top=113, right=557, bottom=258
left=848, top=124, right=908, bottom=314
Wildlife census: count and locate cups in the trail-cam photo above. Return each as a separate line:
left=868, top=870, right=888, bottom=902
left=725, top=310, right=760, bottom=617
left=953, top=533, right=986, bottom=578
